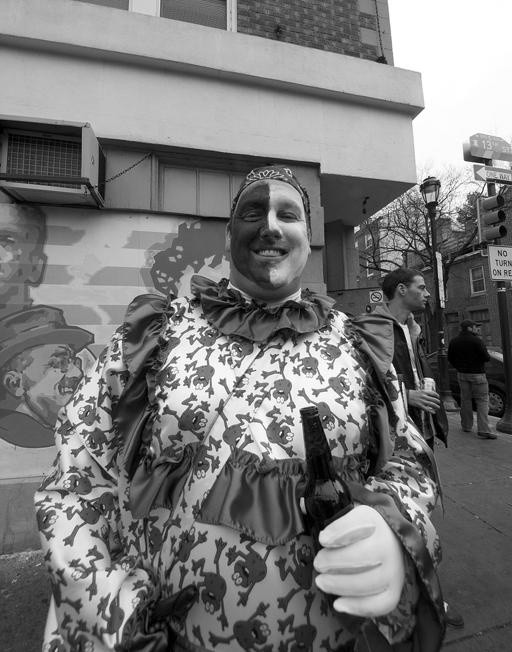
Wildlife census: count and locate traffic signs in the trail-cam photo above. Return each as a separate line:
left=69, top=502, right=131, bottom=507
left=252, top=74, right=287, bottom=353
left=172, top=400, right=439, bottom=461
left=474, top=164, right=512, bottom=185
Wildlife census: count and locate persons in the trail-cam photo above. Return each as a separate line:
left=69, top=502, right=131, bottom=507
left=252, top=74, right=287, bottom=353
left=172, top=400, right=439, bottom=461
left=0, top=304, right=94, bottom=447
left=35, top=163, right=447, bottom=652
left=352, top=267, right=448, bottom=454
left=0, top=200, right=97, bottom=378
left=449, top=319, right=497, bottom=440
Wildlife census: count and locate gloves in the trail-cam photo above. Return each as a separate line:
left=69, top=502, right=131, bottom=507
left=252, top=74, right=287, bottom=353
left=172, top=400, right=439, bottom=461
left=314, top=505, right=408, bottom=618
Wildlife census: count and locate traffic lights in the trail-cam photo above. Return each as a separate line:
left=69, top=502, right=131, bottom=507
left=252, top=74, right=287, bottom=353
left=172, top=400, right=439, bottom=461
left=481, top=195, right=509, bottom=248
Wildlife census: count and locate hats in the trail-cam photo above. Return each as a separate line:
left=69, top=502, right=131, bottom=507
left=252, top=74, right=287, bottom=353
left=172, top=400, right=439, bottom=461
left=460, top=319, right=482, bottom=326
left=232, top=164, right=311, bottom=226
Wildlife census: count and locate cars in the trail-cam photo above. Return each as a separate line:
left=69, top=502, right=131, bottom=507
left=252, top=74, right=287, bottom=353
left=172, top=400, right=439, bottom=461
left=449, top=352, right=504, bottom=419
left=421, top=344, right=504, bottom=389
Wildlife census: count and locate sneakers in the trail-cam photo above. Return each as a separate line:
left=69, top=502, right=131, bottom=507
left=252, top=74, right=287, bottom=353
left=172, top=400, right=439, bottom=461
left=477, top=431, right=497, bottom=438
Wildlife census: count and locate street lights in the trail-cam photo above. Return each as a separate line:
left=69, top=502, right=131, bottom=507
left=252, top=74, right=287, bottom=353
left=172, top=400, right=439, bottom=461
left=418, top=175, right=462, bottom=413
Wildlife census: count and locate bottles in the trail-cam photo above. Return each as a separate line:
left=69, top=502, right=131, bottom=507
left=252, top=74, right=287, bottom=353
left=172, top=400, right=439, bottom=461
left=298, top=404, right=355, bottom=616
